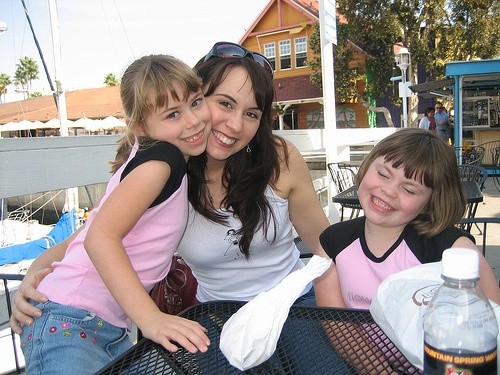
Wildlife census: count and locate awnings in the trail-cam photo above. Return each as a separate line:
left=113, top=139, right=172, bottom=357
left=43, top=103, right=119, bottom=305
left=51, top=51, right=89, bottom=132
left=9, top=42, right=334, bottom=375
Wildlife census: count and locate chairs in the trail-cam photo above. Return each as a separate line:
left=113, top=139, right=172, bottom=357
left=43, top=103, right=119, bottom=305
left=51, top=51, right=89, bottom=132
left=328, top=139, right=500, bottom=257
left=0, top=273, right=27, bottom=375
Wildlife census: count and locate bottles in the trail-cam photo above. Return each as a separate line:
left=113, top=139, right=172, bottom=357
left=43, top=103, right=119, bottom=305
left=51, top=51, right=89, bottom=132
left=424, top=248, right=498, bottom=375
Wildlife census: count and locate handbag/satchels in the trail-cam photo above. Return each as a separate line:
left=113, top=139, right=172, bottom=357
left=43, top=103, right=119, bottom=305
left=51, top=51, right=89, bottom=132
left=149, top=255, right=199, bottom=315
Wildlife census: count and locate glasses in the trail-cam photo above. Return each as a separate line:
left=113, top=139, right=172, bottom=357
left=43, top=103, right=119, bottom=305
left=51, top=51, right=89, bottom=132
left=202, top=42, right=274, bottom=75
left=435, top=106, right=442, bottom=110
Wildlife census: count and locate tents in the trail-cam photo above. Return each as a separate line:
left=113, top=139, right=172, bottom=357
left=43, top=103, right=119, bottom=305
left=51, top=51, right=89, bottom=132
left=0, top=114, right=127, bottom=137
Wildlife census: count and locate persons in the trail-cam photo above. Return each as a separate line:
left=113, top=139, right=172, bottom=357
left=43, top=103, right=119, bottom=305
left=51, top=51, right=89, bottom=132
left=418, top=101, right=454, bottom=145
left=282, top=119, right=292, bottom=130
left=18, top=55, right=210, bottom=375
left=314, top=127, right=500, bottom=375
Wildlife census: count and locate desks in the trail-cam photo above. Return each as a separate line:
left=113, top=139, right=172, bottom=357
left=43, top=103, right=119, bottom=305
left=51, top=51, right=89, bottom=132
left=332, top=180, right=484, bottom=233
left=91, top=300, right=424, bottom=375
